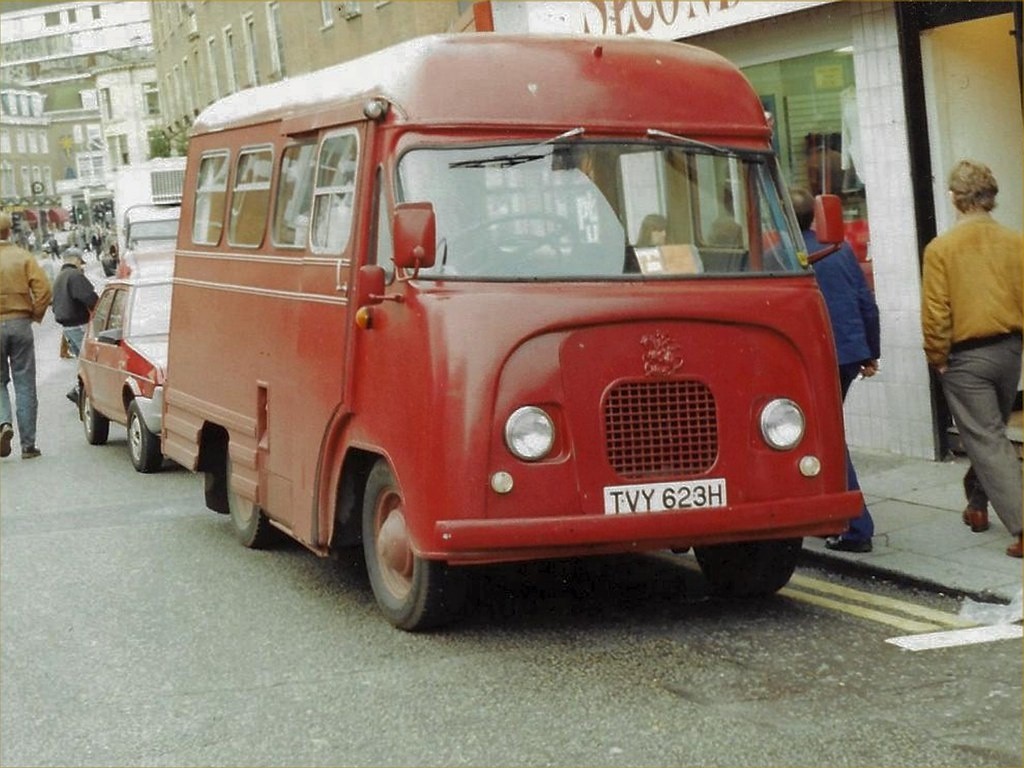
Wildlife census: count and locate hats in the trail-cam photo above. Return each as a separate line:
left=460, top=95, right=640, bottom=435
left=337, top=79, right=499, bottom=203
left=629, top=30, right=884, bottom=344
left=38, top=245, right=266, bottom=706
left=63, top=247, right=86, bottom=264
left=0, top=212, right=13, bottom=230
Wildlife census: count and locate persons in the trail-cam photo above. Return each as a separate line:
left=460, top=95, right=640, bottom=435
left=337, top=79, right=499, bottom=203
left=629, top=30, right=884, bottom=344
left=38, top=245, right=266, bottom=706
left=52, top=248, right=101, bottom=406
left=636, top=214, right=667, bottom=247
left=0, top=214, right=52, bottom=458
left=919, top=161, right=1024, bottom=557
left=13, top=230, right=119, bottom=278
left=707, top=219, right=746, bottom=247
left=763, top=190, right=881, bottom=554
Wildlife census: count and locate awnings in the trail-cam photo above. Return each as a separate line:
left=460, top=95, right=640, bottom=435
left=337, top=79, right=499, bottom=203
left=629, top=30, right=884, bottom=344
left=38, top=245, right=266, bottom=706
left=48, top=208, right=70, bottom=224
left=24, top=208, right=41, bottom=224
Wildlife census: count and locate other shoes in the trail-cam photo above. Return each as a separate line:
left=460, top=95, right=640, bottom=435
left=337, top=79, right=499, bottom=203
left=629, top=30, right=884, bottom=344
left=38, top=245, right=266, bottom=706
left=66, top=388, right=78, bottom=403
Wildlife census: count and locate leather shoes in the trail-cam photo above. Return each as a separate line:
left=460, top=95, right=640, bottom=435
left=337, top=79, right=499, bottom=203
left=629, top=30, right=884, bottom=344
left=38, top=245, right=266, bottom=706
left=963, top=506, right=988, bottom=532
left=1007, top=537, right=1024, bottom=556
left=824, top=535, right=873, bottom=551
left=0, top=425, right=14, bottom=456
left=21, top=446, right=41, bottom=458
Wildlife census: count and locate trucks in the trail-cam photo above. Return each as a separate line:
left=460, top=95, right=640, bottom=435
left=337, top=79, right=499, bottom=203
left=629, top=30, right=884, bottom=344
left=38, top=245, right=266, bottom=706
left=113, top=157, right=188, bottom=261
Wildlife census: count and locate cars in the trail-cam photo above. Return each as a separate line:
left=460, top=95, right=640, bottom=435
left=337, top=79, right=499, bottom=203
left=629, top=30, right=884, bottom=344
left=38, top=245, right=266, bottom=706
left=66, top=245, right=174, bottom=473
left=42, top=231, right=72, bottom=254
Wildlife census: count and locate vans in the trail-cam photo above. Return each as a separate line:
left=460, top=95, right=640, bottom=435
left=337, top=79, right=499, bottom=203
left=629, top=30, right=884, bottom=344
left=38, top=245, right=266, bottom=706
left=161, top=32, right=868, bottom=632
